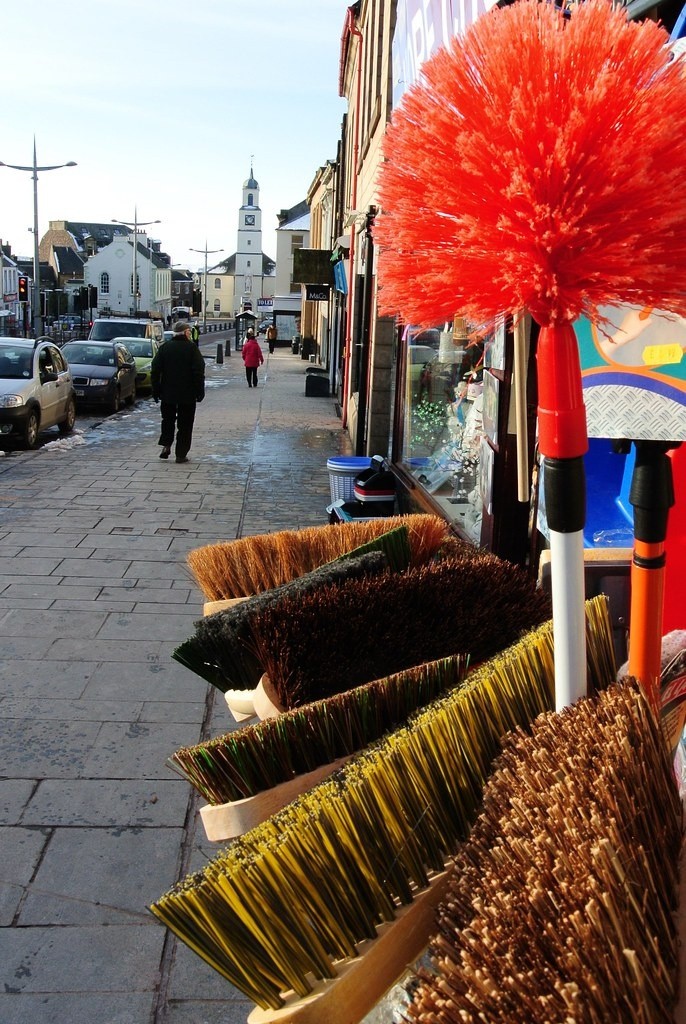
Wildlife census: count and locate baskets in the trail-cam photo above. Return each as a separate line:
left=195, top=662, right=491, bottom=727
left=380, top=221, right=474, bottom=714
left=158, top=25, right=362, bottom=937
left=326, top=456, right=373, bottom=504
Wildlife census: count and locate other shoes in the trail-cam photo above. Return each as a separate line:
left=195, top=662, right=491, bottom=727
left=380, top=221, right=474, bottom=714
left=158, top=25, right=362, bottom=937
left=248, top=384, right=257, bottom=388
left=176, top=456, right=188, bottom=464
left=159, top=444, right=171, bottom=459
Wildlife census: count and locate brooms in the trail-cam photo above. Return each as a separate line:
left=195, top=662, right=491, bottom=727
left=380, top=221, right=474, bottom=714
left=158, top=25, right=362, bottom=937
left=145, top=511, right=685, bottom=1024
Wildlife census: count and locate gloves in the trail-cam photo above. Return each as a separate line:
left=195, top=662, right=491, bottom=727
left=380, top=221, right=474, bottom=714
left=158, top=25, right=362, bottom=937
left=152, top=391, right=161, bottom=403
left=196, top=392, right=204, bottom=402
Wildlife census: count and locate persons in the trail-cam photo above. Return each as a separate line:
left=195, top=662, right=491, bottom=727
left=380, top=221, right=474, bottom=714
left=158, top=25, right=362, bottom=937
left=294, top=316, right=301, bottom=333
left=167, top=314, right=172, bottom=327
left=191, top=321, right=201, bottom=348
left=241, top=336, right=264, bottom=387
left=150, top=321, right=205, bottom=463
left=243, top=328, right=253, bottom=346
left=265, top=325, right=277, bottom=354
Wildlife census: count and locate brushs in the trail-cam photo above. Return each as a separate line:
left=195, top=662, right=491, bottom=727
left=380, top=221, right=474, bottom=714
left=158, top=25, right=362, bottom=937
left=368, top=0, right=686, bottom=718
left=140, top=511, right=686, bottom=1024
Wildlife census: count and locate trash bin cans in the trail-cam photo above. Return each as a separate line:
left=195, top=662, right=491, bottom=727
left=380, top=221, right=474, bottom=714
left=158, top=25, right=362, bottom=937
left=354, top=455, right=398, bottom=515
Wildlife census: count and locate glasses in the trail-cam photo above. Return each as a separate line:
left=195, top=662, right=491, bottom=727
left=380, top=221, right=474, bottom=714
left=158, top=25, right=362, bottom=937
left=295, top=320, right=301, bottom=323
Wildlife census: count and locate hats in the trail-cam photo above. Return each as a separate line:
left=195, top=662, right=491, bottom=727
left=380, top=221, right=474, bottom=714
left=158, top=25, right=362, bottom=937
left=173, top=321, right=190, bottom=333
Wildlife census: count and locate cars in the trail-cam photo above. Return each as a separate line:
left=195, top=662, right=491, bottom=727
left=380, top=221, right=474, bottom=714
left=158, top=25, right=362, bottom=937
left=87, top=316, right=164, bottom=348
left=60, top=340, right=135, bottom=412
left=259, top=320, right=273, bottom=333
left=111, top=336, right=164, bottom=395
left=0, top=336, right=77, bottom=446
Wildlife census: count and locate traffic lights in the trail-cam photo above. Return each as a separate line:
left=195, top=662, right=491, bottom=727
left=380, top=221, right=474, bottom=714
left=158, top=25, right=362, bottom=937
left=18, top=277, right=28, bottom=302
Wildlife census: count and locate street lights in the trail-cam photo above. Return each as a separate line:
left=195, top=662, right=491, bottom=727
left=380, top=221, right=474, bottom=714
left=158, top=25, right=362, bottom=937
left=0, top=133, right=77, bottom=337
left=189, top=240, right=225, bottom=334
left=111, top=204, right=161, bottom=317
